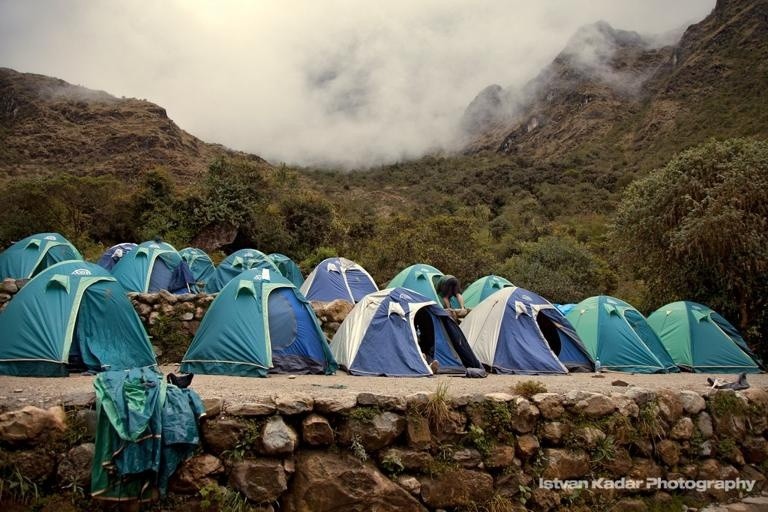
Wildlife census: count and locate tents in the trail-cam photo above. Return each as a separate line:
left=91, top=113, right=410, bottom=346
left=0, top=258, right=167, bottom=381
left=327, top=286, right=490, bottom=377
left=1, top=232, right=525, bottom=313
left=459, top=287, right=598, bottom=375
left=562, top=294, right=683, bottom=374
left=176, top=265, right=343, bottom=379
left=647, top=299, right=766, bottom=373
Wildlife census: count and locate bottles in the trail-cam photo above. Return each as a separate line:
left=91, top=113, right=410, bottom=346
left=594, top=357, right=601, bottom=373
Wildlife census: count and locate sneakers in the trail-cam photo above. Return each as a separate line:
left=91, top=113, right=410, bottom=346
left=430, top=360, right=439, bottom=374
